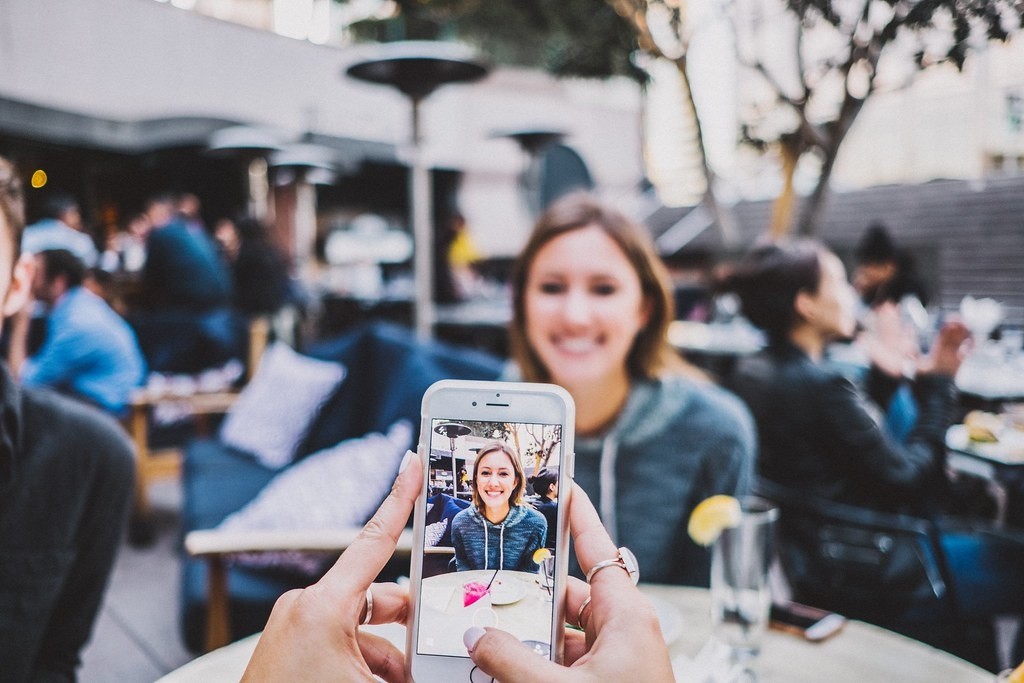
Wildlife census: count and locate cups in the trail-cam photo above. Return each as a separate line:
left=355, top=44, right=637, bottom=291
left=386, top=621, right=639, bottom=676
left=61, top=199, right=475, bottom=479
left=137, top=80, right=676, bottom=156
left=539, top=548, right=555, bottom=602
left=463, top=579, right=492, bottom=619
left=710, top=496, right=779, bottom=659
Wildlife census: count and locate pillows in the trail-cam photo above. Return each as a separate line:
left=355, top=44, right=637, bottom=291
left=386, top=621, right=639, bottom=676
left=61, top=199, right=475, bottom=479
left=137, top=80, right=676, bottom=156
left=217, top=338, right=347, bottom=471
left=210, top=419, right=414, bottom=581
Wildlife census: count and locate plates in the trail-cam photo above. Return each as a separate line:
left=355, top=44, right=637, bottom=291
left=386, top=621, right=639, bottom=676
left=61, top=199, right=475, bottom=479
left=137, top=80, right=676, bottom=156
left=463, top=573, right=527, bottom=605
left=644, top=595, right=682, bottom=645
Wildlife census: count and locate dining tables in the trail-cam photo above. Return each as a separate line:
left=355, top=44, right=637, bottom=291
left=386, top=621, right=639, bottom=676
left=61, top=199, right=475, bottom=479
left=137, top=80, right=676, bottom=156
left=157, top=581, right=1005, bottom=683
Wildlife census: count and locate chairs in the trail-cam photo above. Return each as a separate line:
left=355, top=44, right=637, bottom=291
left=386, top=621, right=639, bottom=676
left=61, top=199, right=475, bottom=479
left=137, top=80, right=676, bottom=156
left=127, top=313, right=271, bottom=548
left=762, top=474, right=996, bottom=674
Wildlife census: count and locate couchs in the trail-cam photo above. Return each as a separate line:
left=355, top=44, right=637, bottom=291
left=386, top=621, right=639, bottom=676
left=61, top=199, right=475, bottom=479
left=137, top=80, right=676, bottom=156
left=181, top=328, right=511, bottom=657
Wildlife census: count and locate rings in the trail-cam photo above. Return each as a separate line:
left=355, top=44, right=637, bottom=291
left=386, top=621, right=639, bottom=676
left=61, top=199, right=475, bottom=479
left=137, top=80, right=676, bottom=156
left=364, top=587, right=373, bottom=626
left=587, top=546, right=640, bottom=587
left=576, top=596, right=597, bottom=631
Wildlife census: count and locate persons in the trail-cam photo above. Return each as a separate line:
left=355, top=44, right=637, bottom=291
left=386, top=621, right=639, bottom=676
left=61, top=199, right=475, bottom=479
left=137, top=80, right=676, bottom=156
left=452, top=441, right=559, bottom=575
left=505, top=190, right=760, bottom=588
left=729, top=220, right=1024, bottom=676
left=2, top=186, right=305, bottom=422
left=242, top=448, right=674, bottom=682
left=0, top=159, right=134, bottom=683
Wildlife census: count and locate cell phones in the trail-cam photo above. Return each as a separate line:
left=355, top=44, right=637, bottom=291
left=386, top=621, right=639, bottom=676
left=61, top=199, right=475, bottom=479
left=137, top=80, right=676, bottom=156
left=405, top=379, right=575, bottom=683
left=722, top=599, right=845, bottom=642
